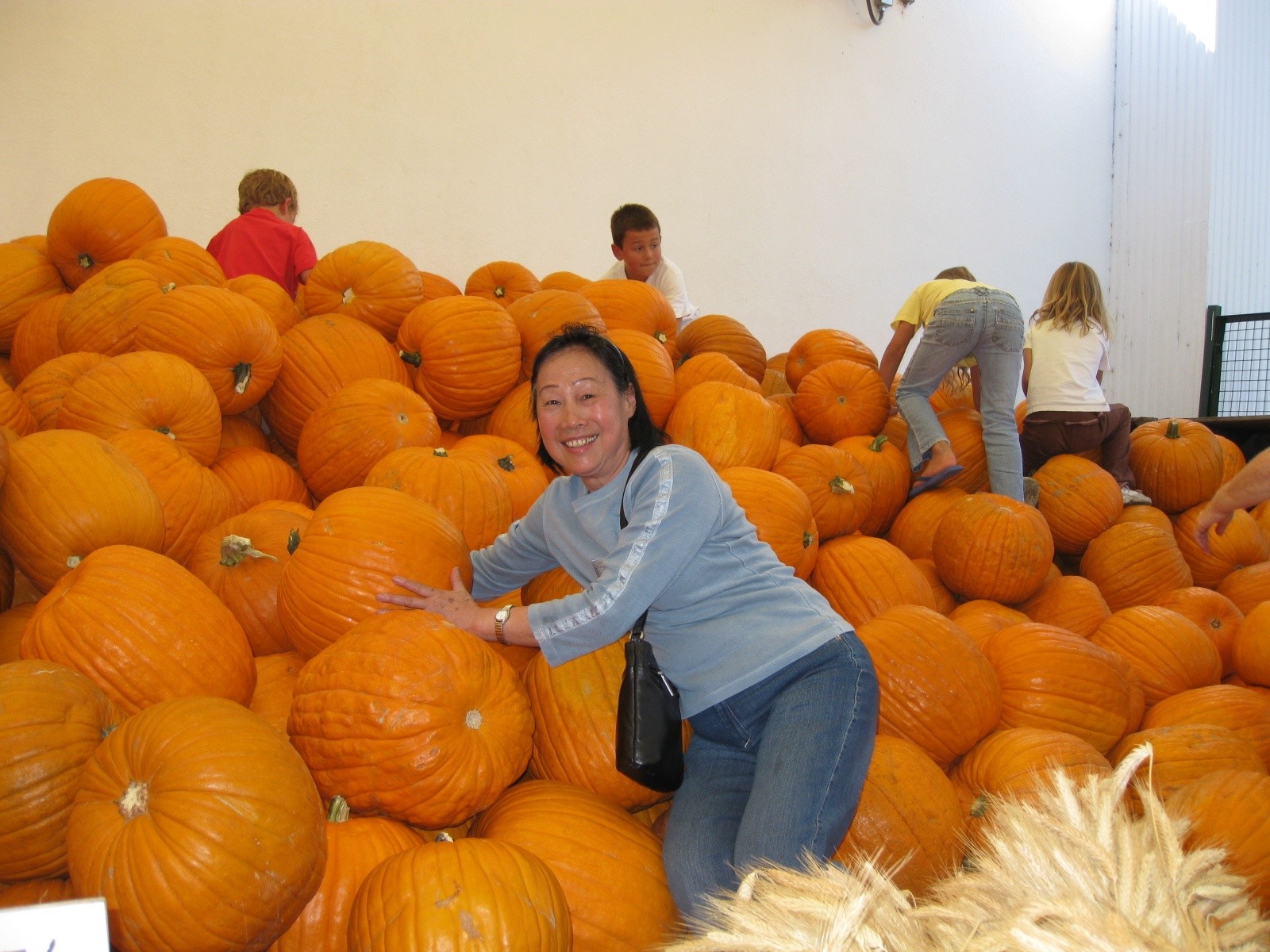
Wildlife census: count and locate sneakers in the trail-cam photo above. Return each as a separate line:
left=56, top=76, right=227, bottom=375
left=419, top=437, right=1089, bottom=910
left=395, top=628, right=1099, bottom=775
left=1023, top=476, right=1038, bottom=507
left=1121, top=487, right=1152, bottom=504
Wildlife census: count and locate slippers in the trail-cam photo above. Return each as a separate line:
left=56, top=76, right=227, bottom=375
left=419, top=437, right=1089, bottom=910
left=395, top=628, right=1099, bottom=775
left=908, top=466, right=963, bottom=501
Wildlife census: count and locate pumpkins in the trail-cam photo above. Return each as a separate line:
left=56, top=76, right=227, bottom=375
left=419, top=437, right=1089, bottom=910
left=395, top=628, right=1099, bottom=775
left=0, top=177, right=1270, bottom=950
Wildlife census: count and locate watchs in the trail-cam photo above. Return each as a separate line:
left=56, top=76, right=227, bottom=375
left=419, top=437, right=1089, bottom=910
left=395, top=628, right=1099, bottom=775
left=495, top=604, right=515, bottom=646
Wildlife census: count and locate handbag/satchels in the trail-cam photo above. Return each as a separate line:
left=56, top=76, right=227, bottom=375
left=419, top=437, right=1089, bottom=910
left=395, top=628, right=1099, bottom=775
left=615, top=641, right=684, bottom=792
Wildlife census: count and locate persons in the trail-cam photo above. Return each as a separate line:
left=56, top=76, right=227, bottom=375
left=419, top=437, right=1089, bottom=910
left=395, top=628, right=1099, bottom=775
left=1018, top=261, right=1154, bottom=505
left=599, top=204, right=701, bottom=334
left=206, top=169, right=318, bottom=302
left=376, top=320, right=878, bottom=935
left=878, top=267, right=1025, bottom=503
